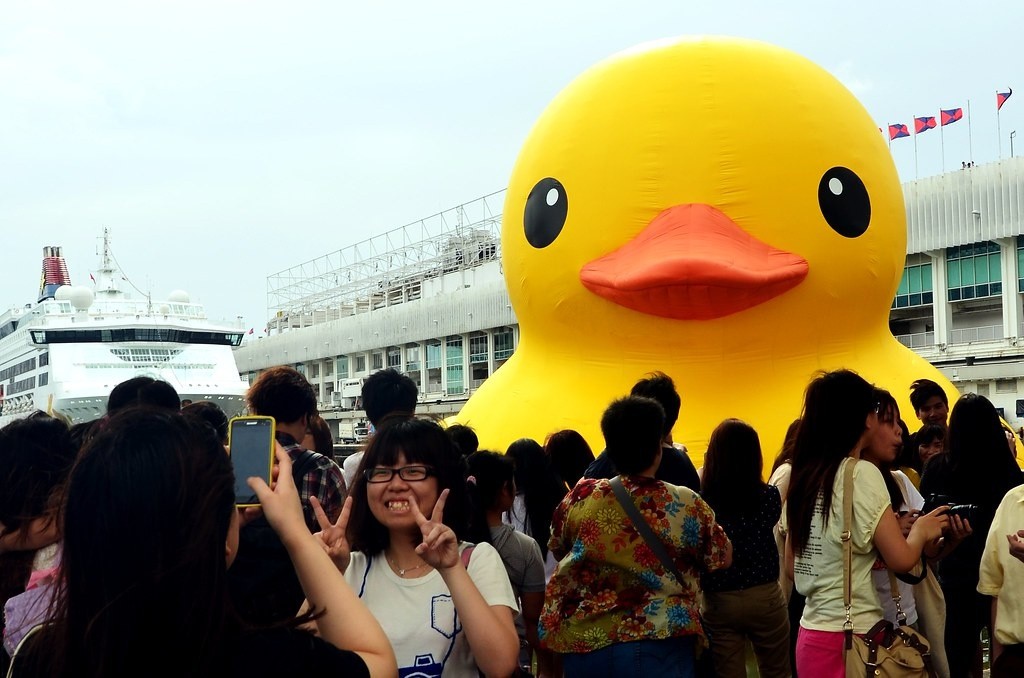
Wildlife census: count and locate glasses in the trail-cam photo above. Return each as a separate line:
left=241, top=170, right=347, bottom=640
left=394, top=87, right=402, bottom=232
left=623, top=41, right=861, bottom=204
left=363, top=465, right=437, bottom=484
left=874, top=402, right=881, bottom=413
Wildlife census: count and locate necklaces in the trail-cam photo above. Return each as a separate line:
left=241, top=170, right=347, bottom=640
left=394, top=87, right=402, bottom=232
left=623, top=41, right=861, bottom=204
left=385, top=545, right=429, bottom=576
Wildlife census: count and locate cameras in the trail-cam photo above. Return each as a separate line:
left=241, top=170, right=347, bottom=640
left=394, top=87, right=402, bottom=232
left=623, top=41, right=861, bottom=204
left=923, top=494, right=982, bottom=532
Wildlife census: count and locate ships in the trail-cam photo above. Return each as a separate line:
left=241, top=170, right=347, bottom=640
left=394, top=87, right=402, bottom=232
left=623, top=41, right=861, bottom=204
left=0, top=224, right=249, bottom=420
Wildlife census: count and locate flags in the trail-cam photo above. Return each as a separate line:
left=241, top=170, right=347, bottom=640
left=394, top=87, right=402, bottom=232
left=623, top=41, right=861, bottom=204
left=914, top=116, right=937, bottom=134
left=940, top=108, right=963, bottom=126
left=995, top=87, right=1013, bottom=111
left=889, top=123, right=911, bottom=140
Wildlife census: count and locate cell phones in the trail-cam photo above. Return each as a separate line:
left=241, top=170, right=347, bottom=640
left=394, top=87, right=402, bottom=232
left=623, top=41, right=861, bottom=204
left=228, top=416, right=276, bottom=507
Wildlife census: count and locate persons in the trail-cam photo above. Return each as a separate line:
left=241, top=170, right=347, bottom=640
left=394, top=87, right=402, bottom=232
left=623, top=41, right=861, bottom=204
left=0, top=367, right=1024, bottom=678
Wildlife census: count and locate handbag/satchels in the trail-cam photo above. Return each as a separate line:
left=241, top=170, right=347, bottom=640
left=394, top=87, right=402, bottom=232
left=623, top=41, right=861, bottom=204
left=844, top=619, right=937, bottom=678
left=698, top=634, right=715, bottom=678
